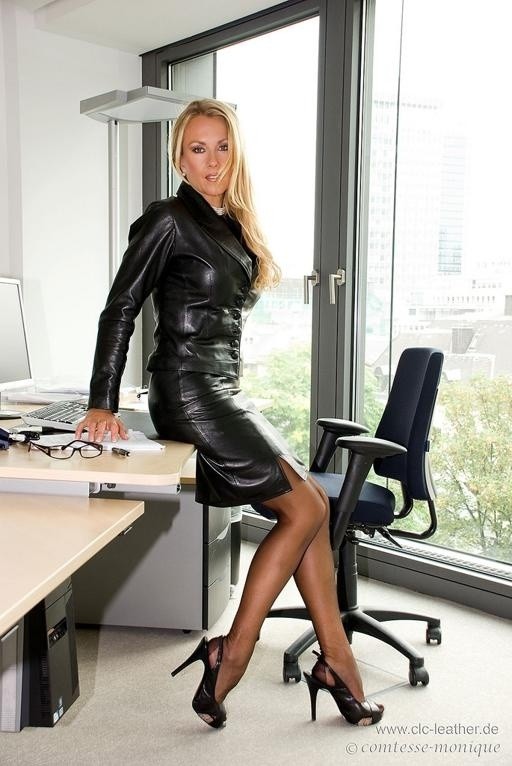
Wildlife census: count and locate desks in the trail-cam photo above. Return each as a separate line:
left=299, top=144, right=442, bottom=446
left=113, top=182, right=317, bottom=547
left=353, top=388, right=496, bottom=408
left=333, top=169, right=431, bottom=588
left=0, top=383, right=243, bottom=635
left=0, top=493, right=147, bottom=734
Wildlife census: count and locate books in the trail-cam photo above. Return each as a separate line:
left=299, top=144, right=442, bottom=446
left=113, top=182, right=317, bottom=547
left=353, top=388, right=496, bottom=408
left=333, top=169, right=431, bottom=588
left=27, top=429, right=166, bottom=453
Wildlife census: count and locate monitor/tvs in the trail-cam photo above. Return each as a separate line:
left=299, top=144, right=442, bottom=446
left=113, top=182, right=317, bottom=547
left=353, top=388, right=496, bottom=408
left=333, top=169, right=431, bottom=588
left=0, top=277, right=36, bottom=418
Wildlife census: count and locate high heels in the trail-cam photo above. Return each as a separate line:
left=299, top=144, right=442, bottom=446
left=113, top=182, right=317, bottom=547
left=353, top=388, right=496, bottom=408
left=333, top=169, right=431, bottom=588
left=171, top=635, right=227, bottom=729
left=303, top=649, right=384, bottom=726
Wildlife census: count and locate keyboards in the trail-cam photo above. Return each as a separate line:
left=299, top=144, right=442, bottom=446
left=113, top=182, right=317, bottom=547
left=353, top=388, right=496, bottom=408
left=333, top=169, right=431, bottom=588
left=21, top=400, right=88, bottom=432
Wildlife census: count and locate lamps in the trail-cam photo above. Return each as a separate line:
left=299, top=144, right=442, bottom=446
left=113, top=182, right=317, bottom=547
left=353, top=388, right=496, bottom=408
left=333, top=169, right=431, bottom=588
left=79, top=87, right=237, bottom=288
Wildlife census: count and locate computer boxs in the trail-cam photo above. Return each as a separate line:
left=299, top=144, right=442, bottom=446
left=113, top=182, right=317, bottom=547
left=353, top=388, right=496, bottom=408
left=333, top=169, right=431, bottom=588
left=30, top=575, right=81, bottom=727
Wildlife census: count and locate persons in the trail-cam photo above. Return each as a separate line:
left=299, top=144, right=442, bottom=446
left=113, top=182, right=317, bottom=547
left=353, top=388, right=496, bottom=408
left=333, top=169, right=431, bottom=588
left=74, top=98, right=385, bottom=729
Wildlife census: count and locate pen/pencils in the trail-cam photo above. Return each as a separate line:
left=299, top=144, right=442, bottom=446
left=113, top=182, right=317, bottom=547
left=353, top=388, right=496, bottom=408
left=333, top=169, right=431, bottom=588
left=112, top=447, right=131, bottom=457
left=16, top=426, right=55, bottom=433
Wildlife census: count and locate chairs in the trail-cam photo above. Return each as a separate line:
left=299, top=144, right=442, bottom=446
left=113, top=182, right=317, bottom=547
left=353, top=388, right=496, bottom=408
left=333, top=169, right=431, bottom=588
left=252, top=348, right=446, bottom=685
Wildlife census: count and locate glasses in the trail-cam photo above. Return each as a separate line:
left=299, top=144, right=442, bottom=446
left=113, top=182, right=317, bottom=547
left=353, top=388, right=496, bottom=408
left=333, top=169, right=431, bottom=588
left=27, top=440, right=103, bottom=460
